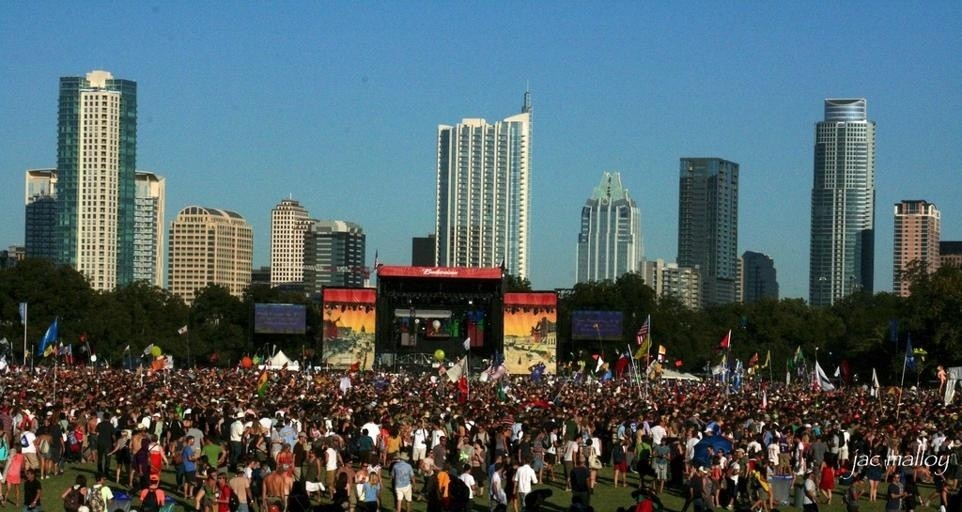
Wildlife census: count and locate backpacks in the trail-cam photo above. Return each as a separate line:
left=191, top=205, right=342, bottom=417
left=541, top=432, right=553, bottom=449
left=141, top=487, right=160, bottom=512
left=613, top=444, right=625, bottom=464
left=87, top=485, right=106, bottom=512
left=222, top=483, right=240, bottom=512
left=843, top=487, right=851, bottom=505
left=63, top=484, right=86, bottom=512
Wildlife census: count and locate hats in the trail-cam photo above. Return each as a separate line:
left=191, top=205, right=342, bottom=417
left=298, top=431, right=309, bottom=439
left=698, top=465, right=707, bottom=473
left=398, top=452, right=410, bottom=460
left=735, top=448, right=746, bottom=454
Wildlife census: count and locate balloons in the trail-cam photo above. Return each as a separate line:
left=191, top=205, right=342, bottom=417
left=152, top=346, right=161, bottom=357
left=434, top=349, right=445, bottom=359
left=242, top=357, right=252, bottom=367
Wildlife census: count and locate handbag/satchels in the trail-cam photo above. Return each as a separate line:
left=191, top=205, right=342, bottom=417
left=587, top=456, right=604, bottom=471
left=39, top=439, right=50, bottom=454
left=173, top=451, right=182, bottom=466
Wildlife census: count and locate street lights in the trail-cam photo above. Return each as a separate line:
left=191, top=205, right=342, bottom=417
left=819, top=274, right=827, bottom=309
left=848, top=274, right=857, bottom=295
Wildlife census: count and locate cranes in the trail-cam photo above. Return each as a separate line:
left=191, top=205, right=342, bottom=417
left=290, top=262, right=376, bottom=288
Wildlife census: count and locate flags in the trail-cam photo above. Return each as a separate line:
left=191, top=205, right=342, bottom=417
left=761, top=350, right=771, bottom=371
left=748, top=353, right=758, bottom=367
left=711, top=330, right=730, bottom=356
left=906, top=333, right=920, bottom=373
left=632, top=336, right=652, bottom=360
left=24, top=340, right=72, bottom=358
left=36, top=318, right=57, bottom=361
left=635, top=316, right=648, bottom=346
left=787, top=345, right=805, bottom=383
left=462, top=337, right=471, bottom=352
left=840, top=357, right=848, bottom=381
left=178, top=324, right=188, bottom=336
left=141, top=342, right=154, bottom=357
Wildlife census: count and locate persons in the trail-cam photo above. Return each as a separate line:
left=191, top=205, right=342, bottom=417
left=1, top=361, right=961, bottom=510
left=428, top=320, right=452, bottom=338
